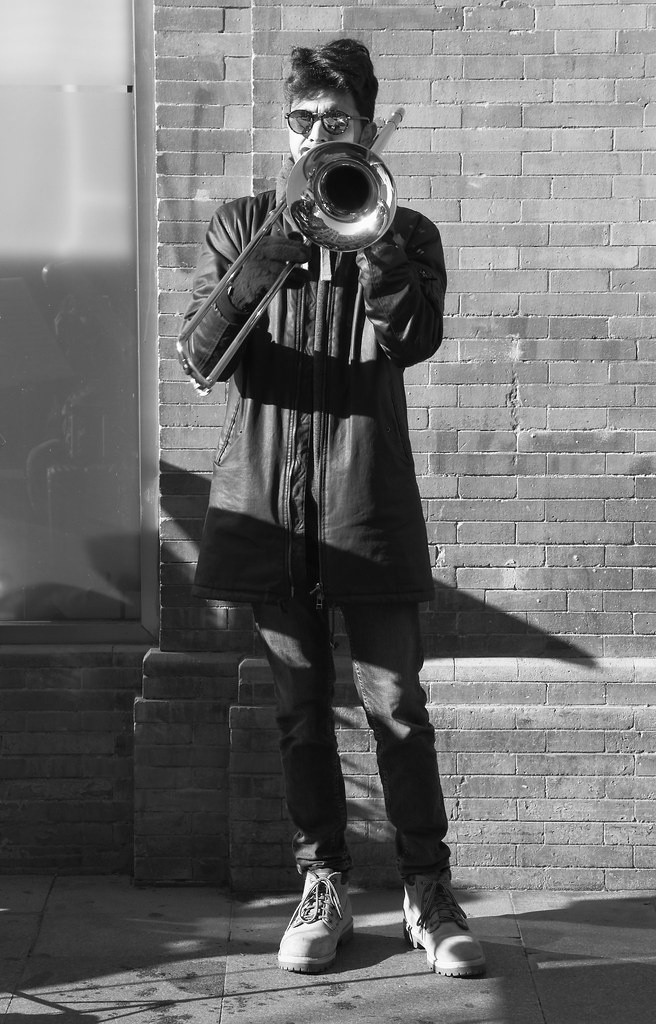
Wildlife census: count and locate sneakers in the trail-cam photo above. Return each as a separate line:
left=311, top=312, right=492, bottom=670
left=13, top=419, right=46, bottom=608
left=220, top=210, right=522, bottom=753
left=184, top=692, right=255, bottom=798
left=403, top=869, right=486, bottom=979
left=277, top=865, right=354, bottom=973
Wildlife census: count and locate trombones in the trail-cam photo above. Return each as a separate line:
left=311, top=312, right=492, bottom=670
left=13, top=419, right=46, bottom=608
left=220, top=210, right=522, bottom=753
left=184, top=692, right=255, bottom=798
left=173, top=106, right=407, bottom=398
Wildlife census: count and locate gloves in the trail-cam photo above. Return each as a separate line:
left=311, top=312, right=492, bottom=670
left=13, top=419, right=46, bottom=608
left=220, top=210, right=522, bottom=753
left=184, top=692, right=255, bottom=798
left=232, top=237, right=309, bottom=310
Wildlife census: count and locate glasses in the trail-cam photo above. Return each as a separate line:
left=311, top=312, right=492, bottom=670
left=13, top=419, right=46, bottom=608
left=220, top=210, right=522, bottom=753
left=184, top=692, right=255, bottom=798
left=285, top=109, right=371, bottom=137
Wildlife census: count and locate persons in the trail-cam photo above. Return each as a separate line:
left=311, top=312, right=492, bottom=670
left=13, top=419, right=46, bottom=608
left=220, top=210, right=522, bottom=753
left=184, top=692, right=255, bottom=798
left=176, top=37, right=486, bottom=977
